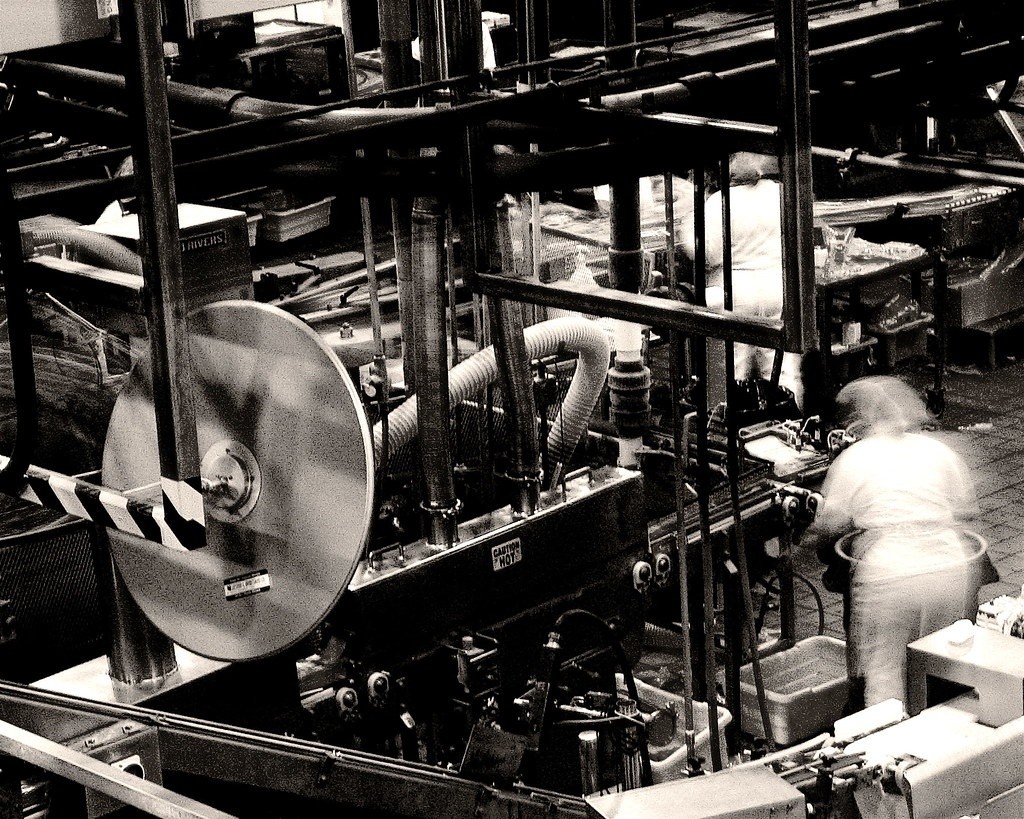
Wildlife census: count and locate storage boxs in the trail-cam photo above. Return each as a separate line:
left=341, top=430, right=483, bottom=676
left=615, top=673, right=732, bottom=784
left=718, top=635, right=850, bottom=744
left=832, top=336, right=878, bottom=382
left=867, top=311, right=934, bottom=367
left=78, top=203, right=253, bottom=340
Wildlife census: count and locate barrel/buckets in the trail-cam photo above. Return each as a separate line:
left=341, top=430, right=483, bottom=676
left=835, top=527, right=989, bottom=714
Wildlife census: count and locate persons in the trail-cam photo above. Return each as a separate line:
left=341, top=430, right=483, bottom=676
left=797, top=375, right=975, bottom=717
left=681, top=150, right=805, bottom=410
